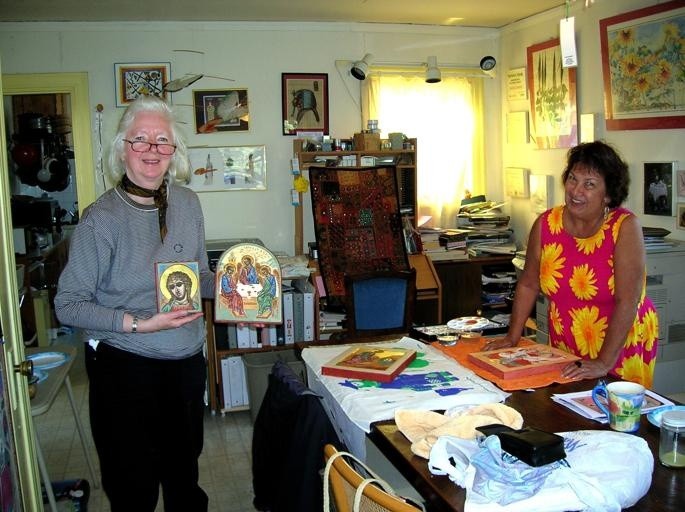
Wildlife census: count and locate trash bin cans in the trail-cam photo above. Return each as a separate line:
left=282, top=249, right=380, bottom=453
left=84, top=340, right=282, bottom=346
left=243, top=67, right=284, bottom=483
left=242, top=349, right=306, bottom=425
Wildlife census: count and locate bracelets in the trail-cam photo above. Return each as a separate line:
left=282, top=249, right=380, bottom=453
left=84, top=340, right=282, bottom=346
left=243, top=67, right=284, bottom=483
left=131, top=316, right=139, bottom=336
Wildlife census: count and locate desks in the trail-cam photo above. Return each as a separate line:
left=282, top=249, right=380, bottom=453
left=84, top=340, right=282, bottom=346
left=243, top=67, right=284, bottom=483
left=301, top=333, right=685, bottom=512
left=430, top=254, right=516, bottom=321
left=24, top=345, right=99, bottom=512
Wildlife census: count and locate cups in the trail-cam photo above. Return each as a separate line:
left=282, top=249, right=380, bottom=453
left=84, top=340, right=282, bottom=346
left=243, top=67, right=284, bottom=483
left=591, top=381, right=646, bottom=433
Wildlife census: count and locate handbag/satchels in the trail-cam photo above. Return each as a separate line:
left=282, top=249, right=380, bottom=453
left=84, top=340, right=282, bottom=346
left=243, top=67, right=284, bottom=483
left=476, top=424, right=566, bottom=467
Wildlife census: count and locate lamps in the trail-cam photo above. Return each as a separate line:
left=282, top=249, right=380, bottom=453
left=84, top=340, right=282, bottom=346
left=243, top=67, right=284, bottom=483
left=335, top=53, right=498, bottom=83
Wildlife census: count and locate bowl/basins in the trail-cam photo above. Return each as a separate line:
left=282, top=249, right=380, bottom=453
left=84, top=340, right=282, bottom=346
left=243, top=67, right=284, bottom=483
left=461, top=329, right=483, bottom=345
left=435, top=333, right=460, bottom=347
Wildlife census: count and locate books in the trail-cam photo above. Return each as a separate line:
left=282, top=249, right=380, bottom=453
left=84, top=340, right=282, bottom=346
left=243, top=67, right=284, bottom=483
left=415, top=199, right=516, bottom=260
left=319, top=311, right=347, bottom=330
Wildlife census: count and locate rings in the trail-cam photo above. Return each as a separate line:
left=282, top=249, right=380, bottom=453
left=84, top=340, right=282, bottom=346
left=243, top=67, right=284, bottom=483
left=581, top=368, right=585, bottom=373
left=575, top=361, right=582, bottom=368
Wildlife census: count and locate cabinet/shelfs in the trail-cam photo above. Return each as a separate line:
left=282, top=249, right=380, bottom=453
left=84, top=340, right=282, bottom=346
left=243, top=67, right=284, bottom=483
left=647, top=251, right=685, bottom=396
left=291, top=137, right=443, bottom=344
left=205, top=253, right=319, bottom=417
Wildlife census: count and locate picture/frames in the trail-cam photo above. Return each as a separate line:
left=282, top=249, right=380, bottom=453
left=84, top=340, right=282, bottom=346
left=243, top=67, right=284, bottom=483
left=641, top=160, right=677, bottom=217
left=599, top=0, right=685, bottom=130
left=676, top=203, right=685, bottom=229
left=192, top=86, right=250, bottom=134
left=186, top=144, right=267, bottom=192
left=114, top=62, right=172, bottom=108
left=527, top=38, right=578, bottom=149
left=321, top=344, right=416, bottom=384
left=282, top=72, right=329, bottom=136
left=468, top=343, right=582, bottom=379
left=154, top=261, right=202, bottom=315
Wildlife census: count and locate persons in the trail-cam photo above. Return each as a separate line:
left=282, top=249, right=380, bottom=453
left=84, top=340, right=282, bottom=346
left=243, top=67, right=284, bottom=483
left=481, top=141, right=659, bottom=390
left=55, top=95, right=217, bottom=512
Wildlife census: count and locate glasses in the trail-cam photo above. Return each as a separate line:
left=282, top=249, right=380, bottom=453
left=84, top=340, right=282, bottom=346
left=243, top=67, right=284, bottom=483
left=122, top=139, right=177, bottom=155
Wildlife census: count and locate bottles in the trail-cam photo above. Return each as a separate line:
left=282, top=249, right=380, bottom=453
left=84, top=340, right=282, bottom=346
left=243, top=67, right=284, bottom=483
left=658, top=411, right=685, bottom=469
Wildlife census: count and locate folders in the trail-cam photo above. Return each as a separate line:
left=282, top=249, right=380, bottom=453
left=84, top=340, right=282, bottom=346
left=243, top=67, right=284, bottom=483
left=227, top=324, right=237, bottom=349
left=277, top=325, right=285, bottom=346
left=236, top=323, right=249, bottom=348
left=304, top=291, right=315, bottom=341
left=270, top=324, right=277, bottom=347
left=227, top=356, right=244, bottom=407
left=261, top=324, right=270, bottom=348
left=242, top=358, right=249, bottom=406
left=283, top=292, right=294, bottom=345
left=220, top=359, right=231, bottom=410
left=249, top=323, right=258, bottom=348
left=293, top=290, right=304, bottom=343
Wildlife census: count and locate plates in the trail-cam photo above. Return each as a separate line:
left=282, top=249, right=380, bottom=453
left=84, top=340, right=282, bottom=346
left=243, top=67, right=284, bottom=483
left=647, top=405, right=685, bottom=429
left=33, top=371, right=49, bottom=383
left=447, top=316, right=489, bottom=330
left=25, top=351, right=70, bottom=370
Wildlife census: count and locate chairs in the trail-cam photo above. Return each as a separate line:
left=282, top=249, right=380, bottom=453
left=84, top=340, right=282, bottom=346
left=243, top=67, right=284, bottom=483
left=318, top=444, right=419, bottom=512
